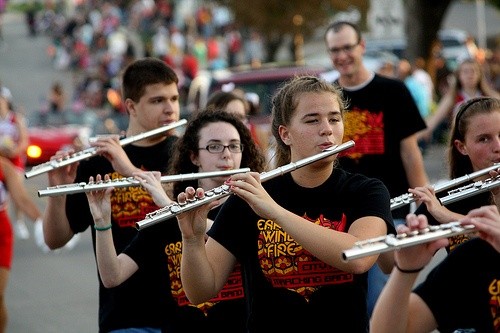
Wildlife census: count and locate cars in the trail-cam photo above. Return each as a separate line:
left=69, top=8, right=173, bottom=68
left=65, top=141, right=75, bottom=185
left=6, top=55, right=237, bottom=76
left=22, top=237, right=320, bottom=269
left=23, top=109, right=82, bottom=163
left=440, top=28, right=471, bottom=71
left=184, top=64, right=322, bottom=170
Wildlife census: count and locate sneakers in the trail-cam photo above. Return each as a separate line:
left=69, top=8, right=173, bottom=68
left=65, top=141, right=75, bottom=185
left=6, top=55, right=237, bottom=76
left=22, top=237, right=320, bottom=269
left=14, top=220, right=29, bottom=240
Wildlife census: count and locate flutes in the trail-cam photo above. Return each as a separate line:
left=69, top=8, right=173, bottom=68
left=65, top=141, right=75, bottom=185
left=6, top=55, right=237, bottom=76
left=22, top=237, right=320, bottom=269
left=24, top=118, right=187, bottom=180
left=340, top=221, right=480, bottom=264
left=135, top=139, right=355, bottom=231
left=37, top=167, right=251, bottom=198
left=390, top=163, right=500, bottom=211
left=438, top=175, right=500, bottom=206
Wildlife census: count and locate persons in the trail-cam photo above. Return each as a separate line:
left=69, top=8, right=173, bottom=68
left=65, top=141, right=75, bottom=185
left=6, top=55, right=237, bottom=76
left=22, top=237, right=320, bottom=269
left=0, top=0, right=500, bottom=333
left=0, top=156, right=42, bottom=333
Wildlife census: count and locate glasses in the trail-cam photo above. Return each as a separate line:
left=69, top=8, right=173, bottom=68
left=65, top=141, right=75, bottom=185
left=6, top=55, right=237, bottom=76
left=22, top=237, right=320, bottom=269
left=198, top=143, right=245, bottom=153
left=327, top=42, right=360, bottom=55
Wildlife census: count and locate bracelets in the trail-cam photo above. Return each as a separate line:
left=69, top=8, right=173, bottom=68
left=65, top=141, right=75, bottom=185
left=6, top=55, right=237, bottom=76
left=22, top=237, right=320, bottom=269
left=95, top=225, right=112, bottom=230
left=394, top=262, right=423, bottom=274
